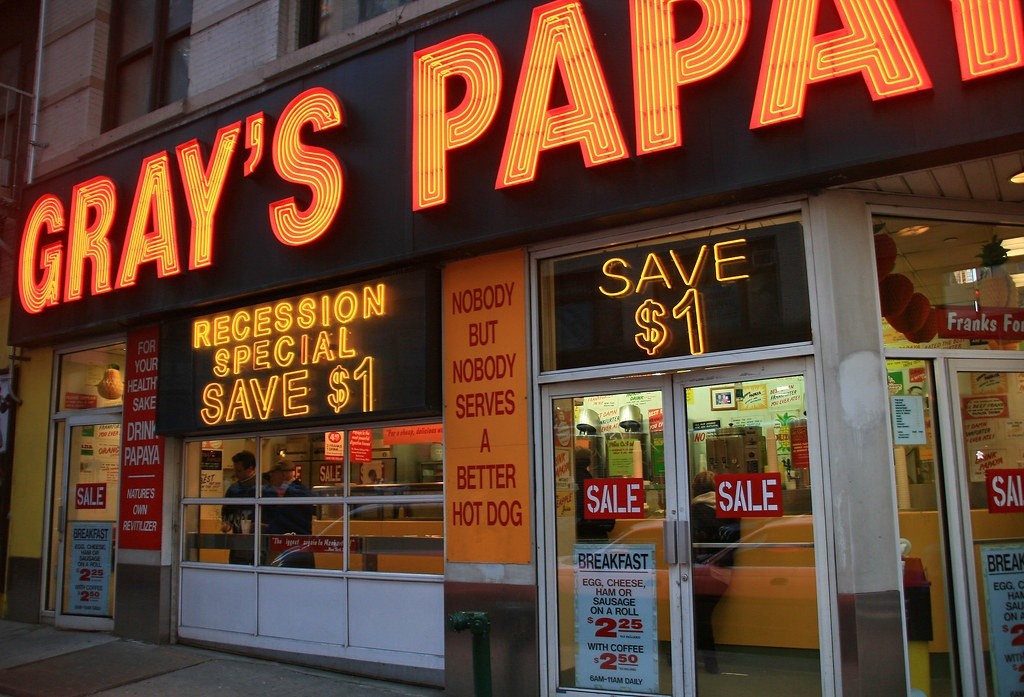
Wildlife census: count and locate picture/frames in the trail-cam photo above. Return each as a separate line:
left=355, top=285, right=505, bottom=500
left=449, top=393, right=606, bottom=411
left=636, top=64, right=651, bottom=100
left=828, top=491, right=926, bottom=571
left=710, top=386, right=738, bottom=411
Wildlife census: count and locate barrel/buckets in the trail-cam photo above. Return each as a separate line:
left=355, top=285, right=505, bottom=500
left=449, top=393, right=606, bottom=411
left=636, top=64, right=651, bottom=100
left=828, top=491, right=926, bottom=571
left=431, top=442, right=442, bottom=461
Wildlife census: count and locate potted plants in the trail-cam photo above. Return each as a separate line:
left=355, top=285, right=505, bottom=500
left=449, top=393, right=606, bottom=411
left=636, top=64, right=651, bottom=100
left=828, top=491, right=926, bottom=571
left=974, top=234, right=1020, bottom=307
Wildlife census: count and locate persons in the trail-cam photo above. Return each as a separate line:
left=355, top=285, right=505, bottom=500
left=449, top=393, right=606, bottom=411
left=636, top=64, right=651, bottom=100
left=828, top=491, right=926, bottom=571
left=666, top=471, right=741, bottom=674
left=717, top=392, right=730, bottom=405
left=219, top=450, right=316, bottom=571
left=574, top=445, right=616, bottom=544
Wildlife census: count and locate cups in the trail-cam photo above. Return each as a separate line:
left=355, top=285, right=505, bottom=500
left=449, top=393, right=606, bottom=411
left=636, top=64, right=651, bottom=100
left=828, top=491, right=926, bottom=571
left=633, top=441, right=643, bottom=478
left=700, top=454, right=706, bottom=471
left=785, top=471, right=796, bottom=490
left=767, top=428, right=779, bottom=472
left=241, top=520, right=251, bottom=534
left=894, top=445, right=911, bottom=510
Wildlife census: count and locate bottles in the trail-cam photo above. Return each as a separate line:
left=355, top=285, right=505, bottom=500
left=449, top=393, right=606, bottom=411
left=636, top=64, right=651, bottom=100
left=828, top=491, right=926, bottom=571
left=399, top=504, right=404, bottom=519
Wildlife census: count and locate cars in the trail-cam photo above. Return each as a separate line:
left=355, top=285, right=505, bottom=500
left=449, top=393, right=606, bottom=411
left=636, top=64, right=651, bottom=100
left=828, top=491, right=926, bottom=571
left=270, top=503, right=444, bottom=571
